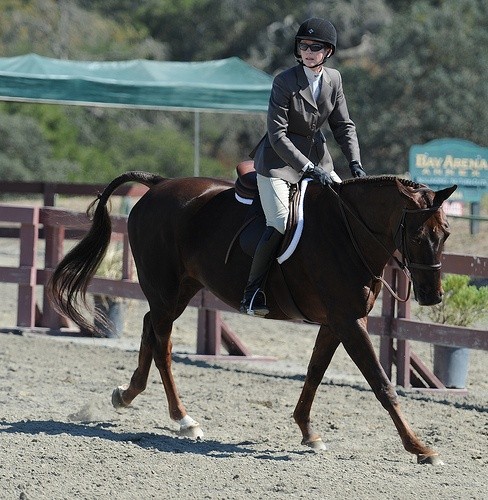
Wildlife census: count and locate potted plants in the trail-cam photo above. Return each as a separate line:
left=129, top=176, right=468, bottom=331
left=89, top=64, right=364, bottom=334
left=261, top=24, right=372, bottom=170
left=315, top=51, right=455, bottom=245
left=415, top=274, right=487, bottom=389
left=92, top=250, right=135, bottom=339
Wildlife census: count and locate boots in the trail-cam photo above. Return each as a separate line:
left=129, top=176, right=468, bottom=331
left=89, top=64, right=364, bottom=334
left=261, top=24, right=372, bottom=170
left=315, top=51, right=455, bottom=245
left=240, top=226, right=285, bottom=315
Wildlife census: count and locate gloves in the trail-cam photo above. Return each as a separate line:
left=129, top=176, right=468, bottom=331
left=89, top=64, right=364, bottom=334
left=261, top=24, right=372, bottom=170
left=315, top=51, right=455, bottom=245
left=349, top=160, right=366, bottom=179
left=304, top=164, right=334, bottom=188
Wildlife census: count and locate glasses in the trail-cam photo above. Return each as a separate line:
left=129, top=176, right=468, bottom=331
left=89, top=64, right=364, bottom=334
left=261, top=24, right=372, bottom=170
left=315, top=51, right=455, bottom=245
left=299, top=40, right=328, bottom=52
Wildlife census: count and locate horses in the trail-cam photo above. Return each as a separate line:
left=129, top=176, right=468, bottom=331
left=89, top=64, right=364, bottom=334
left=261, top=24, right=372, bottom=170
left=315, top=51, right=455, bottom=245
left=47, top=170, right=458, bottom=468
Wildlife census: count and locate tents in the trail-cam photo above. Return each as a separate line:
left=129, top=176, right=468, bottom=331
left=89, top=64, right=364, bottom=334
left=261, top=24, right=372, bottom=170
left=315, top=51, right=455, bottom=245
left=0, top=52, right=275, bottom=176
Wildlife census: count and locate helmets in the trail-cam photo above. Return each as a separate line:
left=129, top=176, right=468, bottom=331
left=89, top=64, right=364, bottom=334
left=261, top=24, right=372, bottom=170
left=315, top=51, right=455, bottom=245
left=295, top=18, right=336, bottom=56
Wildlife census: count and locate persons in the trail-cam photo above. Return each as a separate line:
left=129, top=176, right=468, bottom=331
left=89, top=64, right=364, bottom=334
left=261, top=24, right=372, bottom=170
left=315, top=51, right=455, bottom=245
left=239, top=17, right=366, bottom=318
